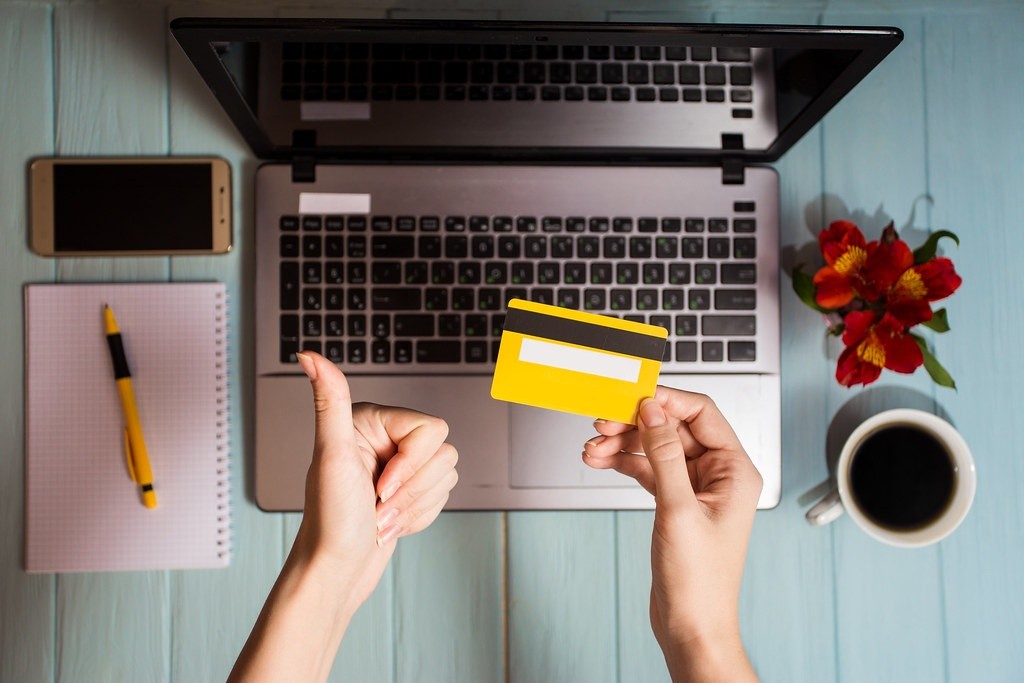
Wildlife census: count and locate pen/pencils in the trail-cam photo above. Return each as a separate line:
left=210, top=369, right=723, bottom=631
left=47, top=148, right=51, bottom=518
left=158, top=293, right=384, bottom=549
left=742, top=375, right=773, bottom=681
left=98, top=299, right=160, bottom=509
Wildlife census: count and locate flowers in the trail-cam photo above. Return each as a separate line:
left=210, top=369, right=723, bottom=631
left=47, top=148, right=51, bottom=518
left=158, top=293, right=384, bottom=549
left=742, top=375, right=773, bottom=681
left=793, top=219, right=963, bottom=396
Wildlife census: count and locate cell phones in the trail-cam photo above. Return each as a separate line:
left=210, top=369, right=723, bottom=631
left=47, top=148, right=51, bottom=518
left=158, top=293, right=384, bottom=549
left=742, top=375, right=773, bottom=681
left=29, top=154, right=232, bottom=255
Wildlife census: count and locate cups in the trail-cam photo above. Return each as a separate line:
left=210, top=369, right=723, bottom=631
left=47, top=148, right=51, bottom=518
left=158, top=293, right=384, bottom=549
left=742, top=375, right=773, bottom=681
left=803, top=407, right=978, bottom=548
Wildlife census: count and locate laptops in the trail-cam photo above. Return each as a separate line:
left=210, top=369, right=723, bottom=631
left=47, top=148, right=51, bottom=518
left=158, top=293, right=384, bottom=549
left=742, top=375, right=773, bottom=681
left=171, top=17, right=905, bottom=511
